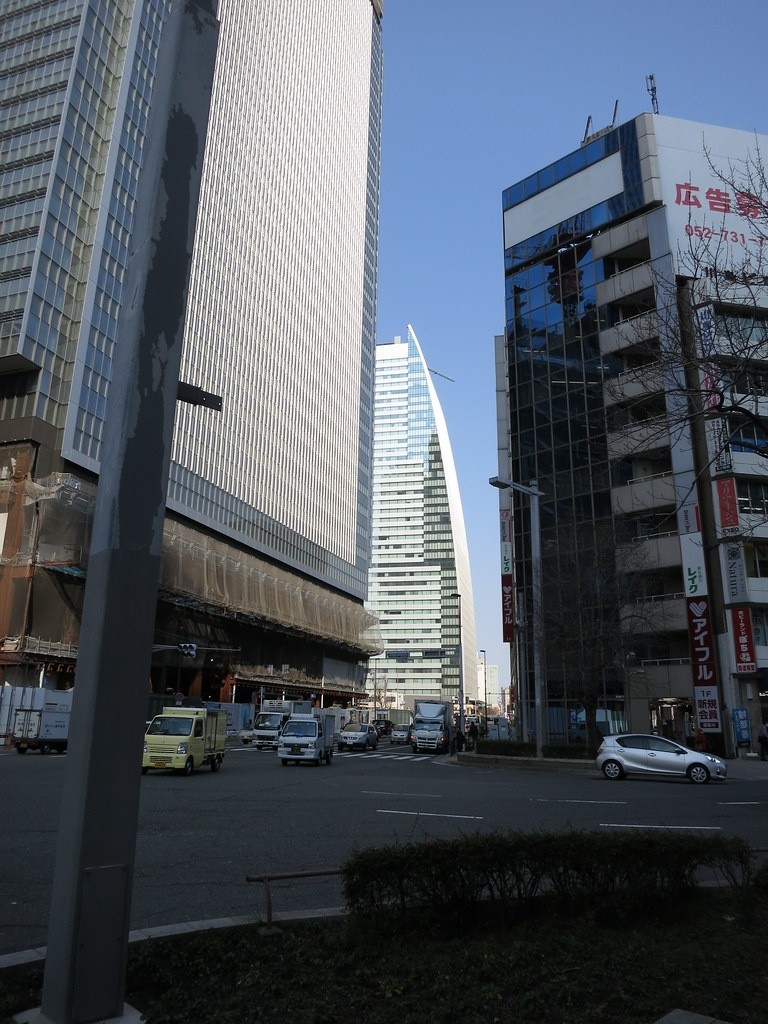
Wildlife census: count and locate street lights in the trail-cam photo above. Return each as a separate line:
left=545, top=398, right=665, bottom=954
left=480, top=649, right=488, bottom=739
left=487, top=474, right=549, bottom=760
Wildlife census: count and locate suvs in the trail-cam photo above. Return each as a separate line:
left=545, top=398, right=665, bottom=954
left=337, top=723, right=378, bottom=751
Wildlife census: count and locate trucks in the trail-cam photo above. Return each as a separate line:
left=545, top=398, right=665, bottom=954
left=409, top=698, right=453, bottom=752
left=11, top=709, right=71, bottom=753
left=372, top=718, right=391, bottom=734
left=140, top=707, right=228, bottom=776
left=275, top=712, right=336, bottom=766
left=252, top=699, right=313, bottom=750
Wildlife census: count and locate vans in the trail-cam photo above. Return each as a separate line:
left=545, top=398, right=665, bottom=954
left=390, top=724, right=412, bottom=744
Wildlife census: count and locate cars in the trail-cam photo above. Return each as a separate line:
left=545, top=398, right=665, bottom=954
left=593, top=733, right=728, bottom=783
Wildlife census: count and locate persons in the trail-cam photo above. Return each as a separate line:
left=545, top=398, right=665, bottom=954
left=508, top=725, right=512, bottom=739
left=759, top=722, right=768, bottom=761
left=452, top=721, right=490, bottom=752
left=695, top=728, right=706, bottom=752
left=349, top=719, right=353, bottom=723
left=245, top=720, right=251, bottom=730
